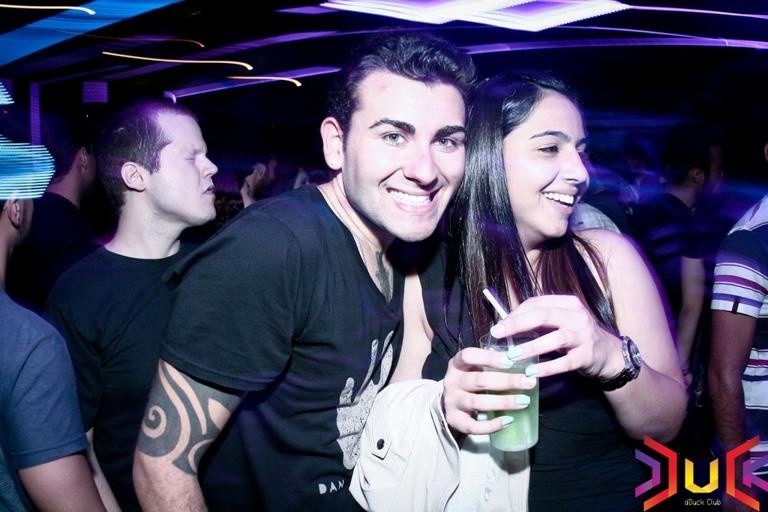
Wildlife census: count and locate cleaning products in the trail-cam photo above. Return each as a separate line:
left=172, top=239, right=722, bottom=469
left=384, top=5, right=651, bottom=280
left=479, top=330, right=541, bottom=453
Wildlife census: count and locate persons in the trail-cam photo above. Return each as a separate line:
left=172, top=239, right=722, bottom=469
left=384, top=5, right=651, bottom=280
left=0, top=133, right=111, bottom=512
left=387, top=68, right=690, bottom=512
left=632, top=137, right=727, bottom=391
left=44, top=100, right=219, bottom=512
left=5, top=130, right=99, bottom=315
left=707, top=145, right=767, bottom=512
left=132, top=38, right=477, bottom=512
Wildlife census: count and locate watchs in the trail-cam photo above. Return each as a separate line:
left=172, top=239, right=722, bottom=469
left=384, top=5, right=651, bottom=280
left=601, top=335, right=643, bottom=392
